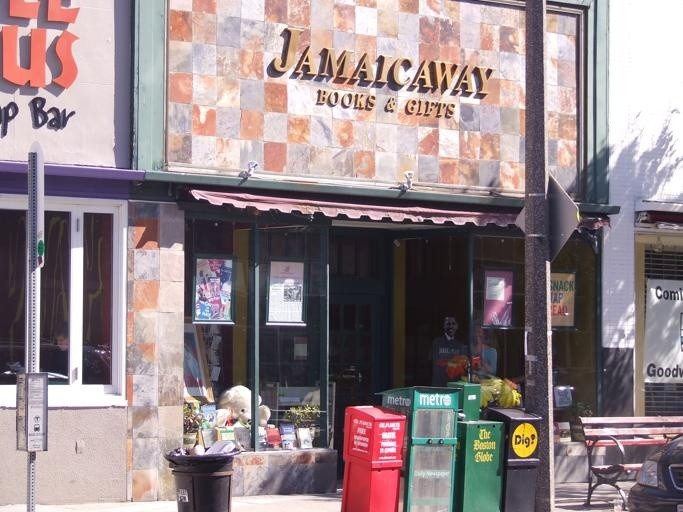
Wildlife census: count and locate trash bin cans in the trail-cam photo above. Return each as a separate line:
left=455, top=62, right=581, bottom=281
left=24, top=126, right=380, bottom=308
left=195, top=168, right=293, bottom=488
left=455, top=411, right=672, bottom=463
left=162, top=445, right=242, bottom=512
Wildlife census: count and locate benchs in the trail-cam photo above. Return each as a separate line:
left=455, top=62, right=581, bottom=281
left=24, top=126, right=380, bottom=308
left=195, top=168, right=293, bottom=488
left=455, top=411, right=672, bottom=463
left=579, top=416, right=683, bottom=511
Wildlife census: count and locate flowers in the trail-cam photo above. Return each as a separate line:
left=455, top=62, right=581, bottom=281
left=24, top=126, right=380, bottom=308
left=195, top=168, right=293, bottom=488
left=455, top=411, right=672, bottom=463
left=284, top=404, right=321, bottom=427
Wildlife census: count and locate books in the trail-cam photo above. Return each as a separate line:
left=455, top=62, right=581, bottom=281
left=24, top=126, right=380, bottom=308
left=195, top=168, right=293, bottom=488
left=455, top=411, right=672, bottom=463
left=201, top=423, right=313, bottom=452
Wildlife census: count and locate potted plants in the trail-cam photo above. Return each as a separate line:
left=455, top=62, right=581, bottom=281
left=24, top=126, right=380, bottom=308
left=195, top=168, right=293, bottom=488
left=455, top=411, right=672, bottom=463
left=569, top=401, right=592, bottom=442
left=183, top=406, right=208, bottom=447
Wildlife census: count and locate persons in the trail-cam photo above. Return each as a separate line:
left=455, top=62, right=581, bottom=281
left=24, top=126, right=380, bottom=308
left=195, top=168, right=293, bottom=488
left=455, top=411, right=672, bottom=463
left=466, top=318, right=497, bottom=382
left=428, top=314, right=467, bottom=387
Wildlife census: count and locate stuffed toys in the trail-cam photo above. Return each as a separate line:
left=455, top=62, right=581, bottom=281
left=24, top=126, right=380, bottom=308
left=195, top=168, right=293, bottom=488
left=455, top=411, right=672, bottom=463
left=216, top=384, right=275, bottom=451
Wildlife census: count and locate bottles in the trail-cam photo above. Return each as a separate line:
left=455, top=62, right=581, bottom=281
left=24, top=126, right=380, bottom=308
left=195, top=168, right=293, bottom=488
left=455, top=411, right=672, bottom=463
left=193, top=429, right=205, bottom=456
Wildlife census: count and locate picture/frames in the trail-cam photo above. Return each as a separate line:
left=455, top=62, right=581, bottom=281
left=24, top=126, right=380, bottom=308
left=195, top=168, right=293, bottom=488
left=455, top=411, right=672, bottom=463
left=185, top=318, right=215, bottom=405
